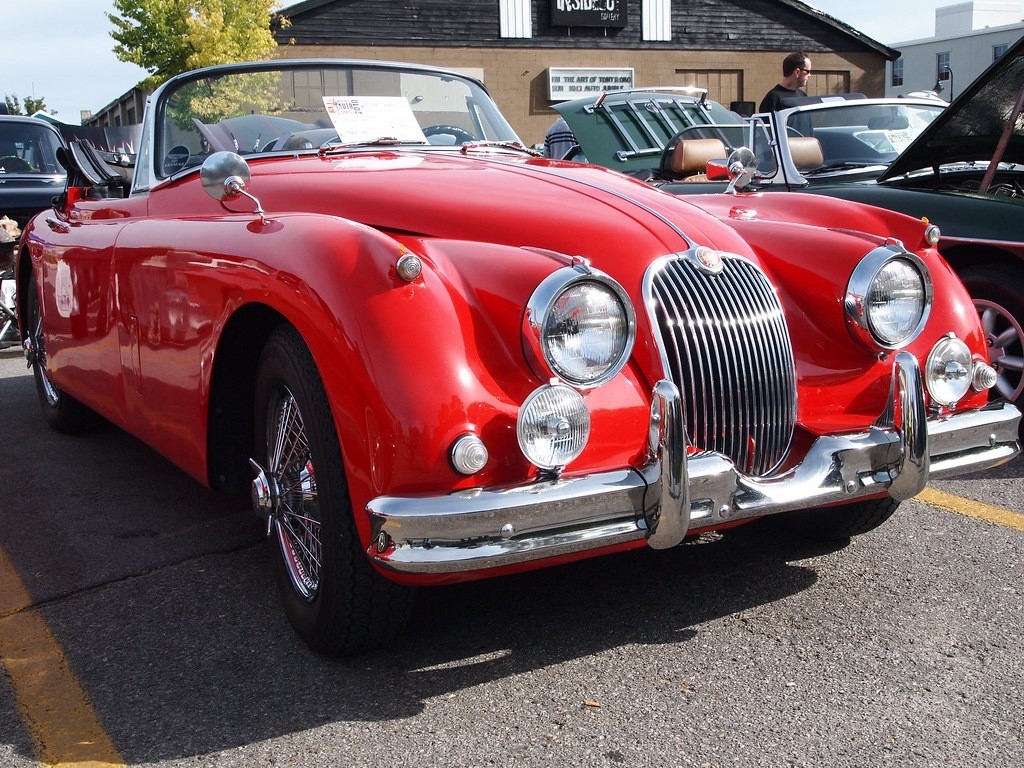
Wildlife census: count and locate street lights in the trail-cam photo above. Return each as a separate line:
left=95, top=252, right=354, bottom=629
left=933, top=67, right=953, bottom=105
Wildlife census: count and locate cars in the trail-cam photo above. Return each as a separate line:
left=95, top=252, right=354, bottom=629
left=0, top=110, right=69, bottom=219
left=14, top=59, right=1023, bottom=652
left=545, top=40, right=1024, bottom=428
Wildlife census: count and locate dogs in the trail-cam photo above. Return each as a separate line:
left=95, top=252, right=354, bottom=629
left=0, top=215, right=22, bottom=243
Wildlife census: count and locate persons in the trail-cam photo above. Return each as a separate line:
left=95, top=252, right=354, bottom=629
left=758, top=52, right=814, bottom=138
left=543, top=118, right=589, bottom=164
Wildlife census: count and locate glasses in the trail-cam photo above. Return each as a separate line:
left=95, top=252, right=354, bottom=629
left=799, top=68, right=810, bottom=74
